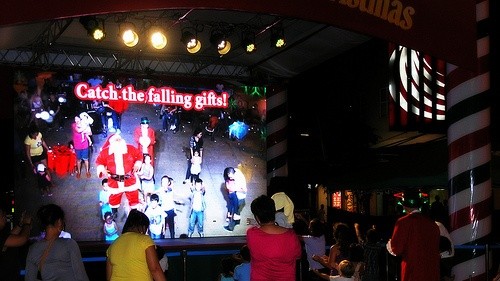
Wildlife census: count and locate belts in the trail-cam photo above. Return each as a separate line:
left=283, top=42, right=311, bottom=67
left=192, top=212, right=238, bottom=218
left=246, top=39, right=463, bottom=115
left=110, top=174, right=129, bottom=182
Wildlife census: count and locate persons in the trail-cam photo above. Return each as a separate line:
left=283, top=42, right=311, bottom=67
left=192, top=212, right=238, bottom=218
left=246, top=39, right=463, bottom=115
left=220, top=191, right=455, bottom=280
left=0, top=66, right=267, bottom=242
left=105, top=208, right=166, bottom=280
left=0, top=203, right=89, bottom=280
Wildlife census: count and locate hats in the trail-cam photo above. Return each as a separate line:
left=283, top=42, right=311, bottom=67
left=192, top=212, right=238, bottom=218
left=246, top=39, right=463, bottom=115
left=399, top=188, right=421, bottom=208
left=139, top=116, right=149, bottom=124
left=99, top=133, right=122, bottom=149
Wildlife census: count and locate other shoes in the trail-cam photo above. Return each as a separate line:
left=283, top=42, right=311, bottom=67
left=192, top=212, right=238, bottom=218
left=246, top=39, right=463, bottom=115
left=75, top=173, right=81, bottom=178
left=226, top=216, right=240, bottom=224
left=182, top=178, right=189, bottom=184
left=41, top=191, right=55, bottom=198
left=86, top=172, right=91, bottom=178
left=223, top=226, right=233, bottom=231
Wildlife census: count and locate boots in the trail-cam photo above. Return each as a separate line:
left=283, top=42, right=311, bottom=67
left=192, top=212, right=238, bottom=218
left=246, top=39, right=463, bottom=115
left=111, top=208, right=117, bottom=220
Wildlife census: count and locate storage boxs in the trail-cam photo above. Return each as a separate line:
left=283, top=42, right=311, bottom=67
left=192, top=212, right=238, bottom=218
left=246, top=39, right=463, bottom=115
left=47, top=145, right=78, bottom=176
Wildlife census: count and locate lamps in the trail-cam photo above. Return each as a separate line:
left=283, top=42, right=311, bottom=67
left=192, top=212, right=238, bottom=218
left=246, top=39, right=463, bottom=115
left=87, top=16, right=108, bottom=41
left=179, top=26, right=202, bottom=54
left=237, top=31, right=256, bottom=54
left=210, top=26, right=233, bottom=55
left=268, top=30, right=287, bottom=50
left=116, top=21, right=139, bottom=48
left=148, top=25, right=168, bottom=49
